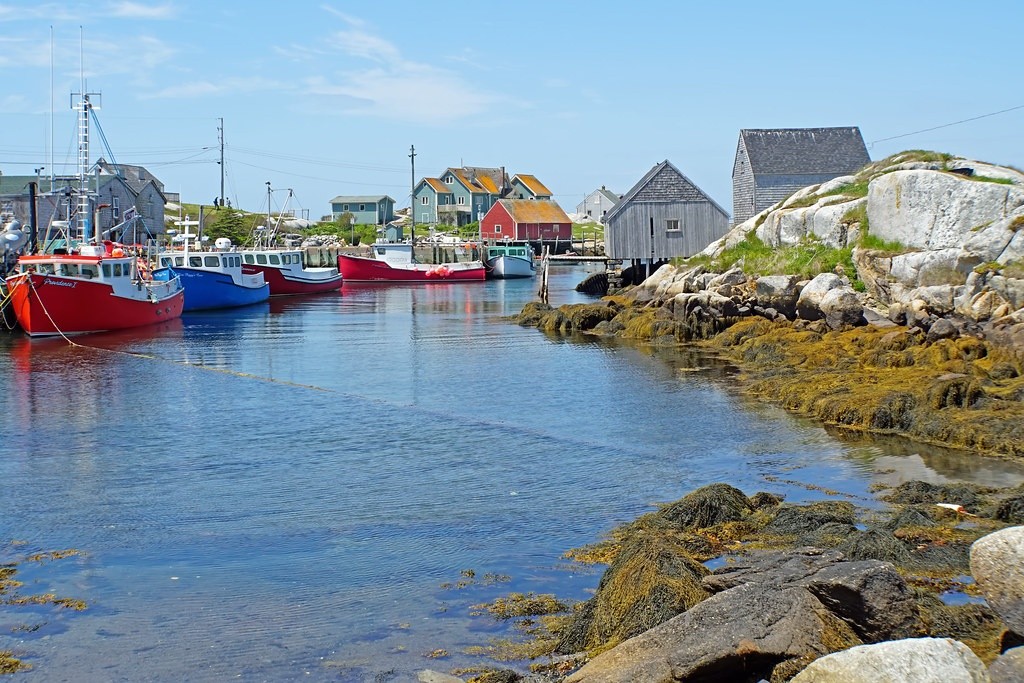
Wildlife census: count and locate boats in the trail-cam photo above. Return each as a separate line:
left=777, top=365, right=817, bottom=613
left=242, top=181, right=343, bottom=295
left=336, top=193, right=486, bottom=282
left=479, top=195, right=540, bottom=280
left=5, top=24, right=185, bottom=339
left=154, top=205, right=271, bottom=313
